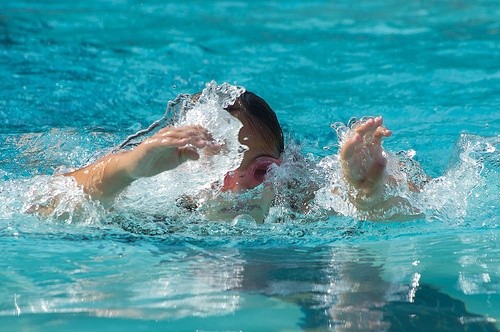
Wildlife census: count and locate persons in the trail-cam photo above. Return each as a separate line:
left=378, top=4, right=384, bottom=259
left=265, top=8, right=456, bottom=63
left=26, top=86, right=426, bottom=226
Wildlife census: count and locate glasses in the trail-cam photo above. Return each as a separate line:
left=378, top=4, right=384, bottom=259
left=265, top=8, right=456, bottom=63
left=211, top=156, right=281, bottom=191
